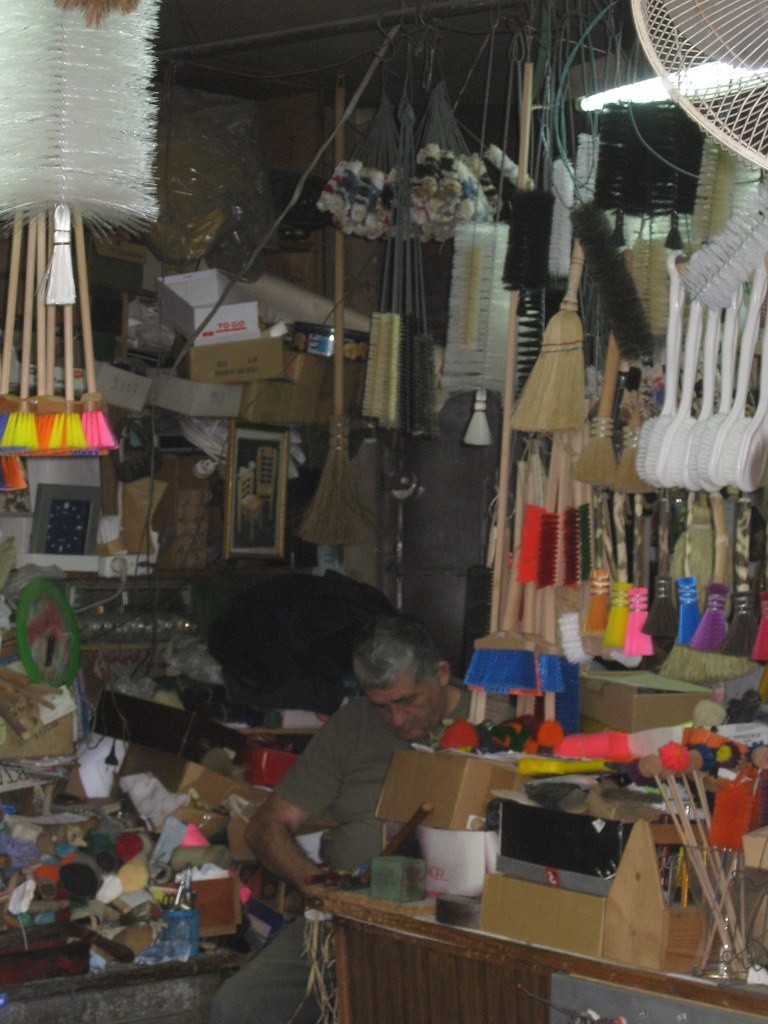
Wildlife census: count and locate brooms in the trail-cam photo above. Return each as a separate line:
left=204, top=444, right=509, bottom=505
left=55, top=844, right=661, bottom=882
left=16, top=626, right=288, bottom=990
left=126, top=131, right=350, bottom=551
left=468, top=196, right=767, bottom=694
left=0, top=210, right=126, bottom=490
left=298, top=83, right=382, bottom=545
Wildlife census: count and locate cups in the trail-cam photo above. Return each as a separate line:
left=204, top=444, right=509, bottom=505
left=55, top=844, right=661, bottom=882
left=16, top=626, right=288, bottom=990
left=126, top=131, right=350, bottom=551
left=683, top=845, right=748, bottom=982
left=164, top=911, right=200, bottom=959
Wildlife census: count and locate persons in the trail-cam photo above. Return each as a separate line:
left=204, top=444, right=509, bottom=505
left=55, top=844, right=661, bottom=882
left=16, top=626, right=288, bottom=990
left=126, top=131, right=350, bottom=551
left=208, top=614, right=515, bottom=1023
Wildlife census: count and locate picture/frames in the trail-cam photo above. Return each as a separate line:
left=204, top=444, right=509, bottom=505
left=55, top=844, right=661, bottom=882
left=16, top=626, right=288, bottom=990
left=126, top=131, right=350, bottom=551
left=28, top=484, right=104, bottom=555
left=221, top=419, right=291, bottom=561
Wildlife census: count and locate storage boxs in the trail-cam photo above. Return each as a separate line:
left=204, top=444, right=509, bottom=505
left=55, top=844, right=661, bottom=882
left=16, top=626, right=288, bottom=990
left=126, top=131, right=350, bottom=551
left=145, top=367, right=243, bottom=418
left=109, top=742, right=268, bottom=816
left=88, top=238, right=148, bottom=288
left=577, top=670, right=712, bottom=732
left=89, top=691, right=244, bottom=762
left=0, top=686, right=75, bottom=761
left=241, top=380, right=319, bottom=426
left=96, top=88, right=258, bottom=274
left=228, top=798, right=332, bottom=920
left=286, top=350, right=359, bottom=425
left=373, top=749, right=535, bottom=829
left=151, top=806, right=228, bottom=836
left=480, top=818, right=672, bottom=965
left=114, top=453, right=209, bottom=571
left=240, top=897, right=296, bottom=948
left=95, top=360, right=151, bottom=411
left=0, top=329, right=89, bottom=391
left=172, top=335, right=286, bottom=384
left=160, top=875, right=243, bottom=937
left=155, top=269, right=261, bottom=347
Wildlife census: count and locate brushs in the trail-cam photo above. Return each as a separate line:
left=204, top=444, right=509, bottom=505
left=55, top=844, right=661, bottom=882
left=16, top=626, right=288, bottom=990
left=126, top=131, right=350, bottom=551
left=1, top=1, right=162, bottom=308
left=502, top=0, right=768, bottom=493
left=462, top=431, right=568, bottom=698
left=315, top=80, right=535, bottom=243
left=345, top=100, right=538, bottom=447
left=514, top=491, right=768, bottom=664
left=626, top=698, right=768, bottom=973
left=1, top=205, right=120, bottom=493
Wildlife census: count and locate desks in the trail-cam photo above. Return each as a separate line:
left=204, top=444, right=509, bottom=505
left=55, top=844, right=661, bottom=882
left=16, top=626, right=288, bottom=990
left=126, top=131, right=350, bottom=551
left=303, top=881, right=768, bottom=1023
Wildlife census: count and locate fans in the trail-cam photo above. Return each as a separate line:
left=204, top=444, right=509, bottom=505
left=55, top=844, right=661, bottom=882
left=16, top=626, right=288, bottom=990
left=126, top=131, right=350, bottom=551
left=630, top=1, right=768, bottom=177
